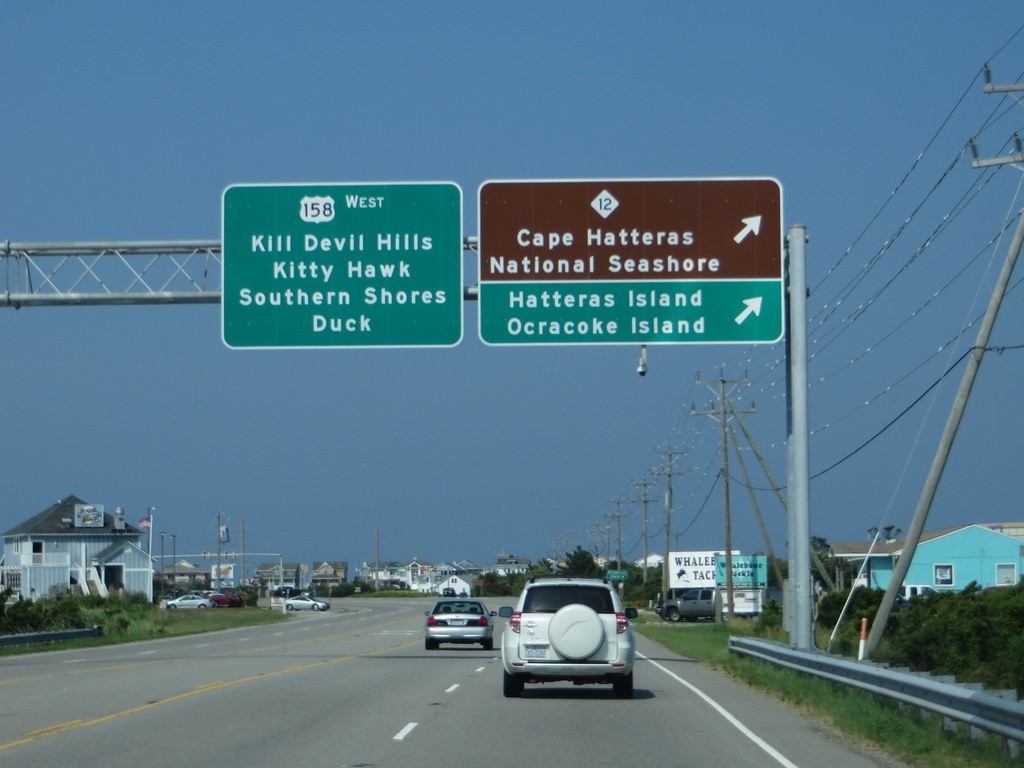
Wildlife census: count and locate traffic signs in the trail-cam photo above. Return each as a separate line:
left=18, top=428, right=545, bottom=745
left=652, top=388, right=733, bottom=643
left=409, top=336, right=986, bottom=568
left=477, top=176, right=784, bottom=282
left=476, top=281, right=786, bottom=346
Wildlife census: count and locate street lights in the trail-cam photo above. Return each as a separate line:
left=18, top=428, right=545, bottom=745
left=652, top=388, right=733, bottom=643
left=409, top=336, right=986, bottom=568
left=169, top=535, right=176, bottom=600
left=147, top=505, right=157, bottom=553
left=160, top=532, right=169, bottom=603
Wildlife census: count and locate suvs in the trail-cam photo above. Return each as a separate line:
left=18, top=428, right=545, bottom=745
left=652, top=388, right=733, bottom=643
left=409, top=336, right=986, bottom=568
left=498, top=577, right=639, bottom=697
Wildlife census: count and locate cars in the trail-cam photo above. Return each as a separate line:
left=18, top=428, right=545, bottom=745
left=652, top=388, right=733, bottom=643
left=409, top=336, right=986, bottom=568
left=423, top=598, right=498, bottom=650
left=283, top=595, right=330, bottom=612
left=193, top=590, right=244, bottom=608
left=654, top=588, right=716, bottom=622
left=444, top=587, right=457, bottom=598
left=165, top=594, right=216, bottom=610
